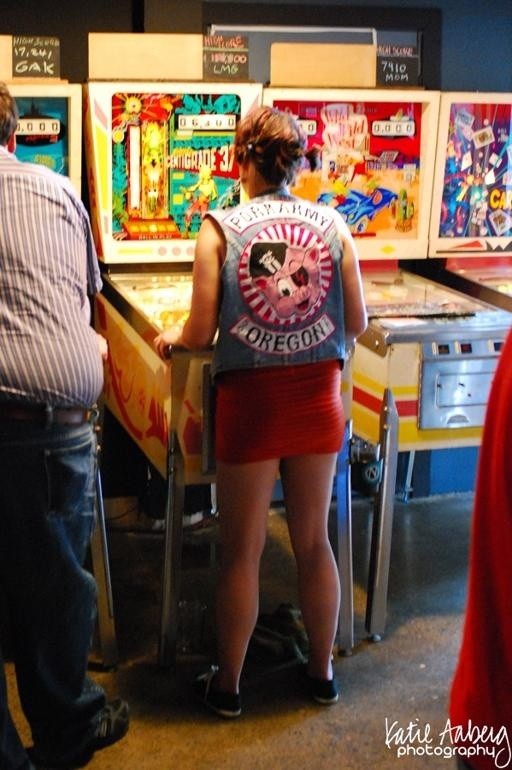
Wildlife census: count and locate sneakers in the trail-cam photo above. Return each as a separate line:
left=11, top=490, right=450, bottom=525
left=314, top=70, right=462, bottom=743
left=304, top=666, right=339, bottom=705
left=191, top=674, right=242, bottom=718
left=39, top=697, right=129, bottom=769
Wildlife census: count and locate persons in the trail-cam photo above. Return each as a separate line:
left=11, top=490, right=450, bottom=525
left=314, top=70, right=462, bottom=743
left=151, top=107, right=370, bottom=720
left=0, top=79, right=134, bottom=767
left=445, top=326, right=511, bottom=770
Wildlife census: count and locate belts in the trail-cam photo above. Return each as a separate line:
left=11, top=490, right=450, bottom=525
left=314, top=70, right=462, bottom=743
left=2, top=403, right=83, bottom=425
